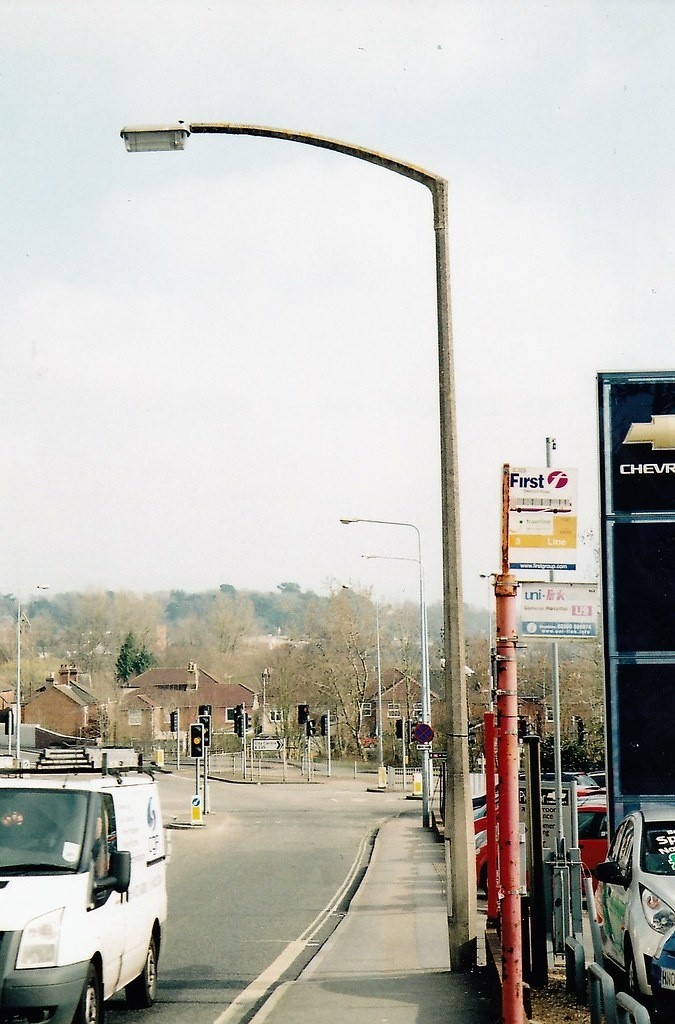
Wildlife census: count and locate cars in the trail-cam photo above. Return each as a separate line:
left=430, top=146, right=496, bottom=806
left=589, top=805, right=675, bottom=1024
left=473, top=773, right=610, bottom=896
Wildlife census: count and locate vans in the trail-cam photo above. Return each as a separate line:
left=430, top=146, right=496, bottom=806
left=0, top=766, right=168, bottom=1023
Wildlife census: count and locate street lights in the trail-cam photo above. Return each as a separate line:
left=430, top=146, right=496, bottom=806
left=338, top=516, right=431, bottom=827
left=16, top=584, right=50, bottom=761
left=117, top=119, right=474, bottom=974
left=341, top=583, right=388, bottom=789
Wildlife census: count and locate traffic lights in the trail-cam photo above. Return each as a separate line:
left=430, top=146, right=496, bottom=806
left=245, top=712, right=252, bottom=730
left=170, top=711, right=177, bottom=732
left=237, top=715, right=244, bottom=737
left=198, top=704, right=211, bottom=715
left=298, top=705, right=309, bottom=724
left=233, top=704, right=241, bottom=734
left=394, top=720, right=403, bottom=740
left=197, top=715, right=212, bottom=748
left=321, top=714, right=329, bottom=736
left=189, top=724, right=204, bottom=759
